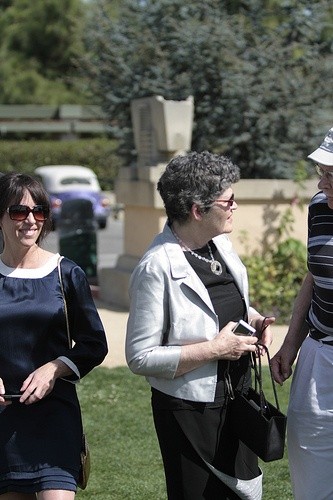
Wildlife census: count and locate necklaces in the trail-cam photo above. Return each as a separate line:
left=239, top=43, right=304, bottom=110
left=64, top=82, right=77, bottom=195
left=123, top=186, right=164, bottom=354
left=170, top=226, right=222, bottom=276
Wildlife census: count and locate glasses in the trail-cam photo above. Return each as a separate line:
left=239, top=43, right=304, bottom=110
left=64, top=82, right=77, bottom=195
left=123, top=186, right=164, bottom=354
left=209, top=193, right=234, bottom=206
left=7, top=205, right=49, bottom=221
left=314, top=164, right=333, bottom=181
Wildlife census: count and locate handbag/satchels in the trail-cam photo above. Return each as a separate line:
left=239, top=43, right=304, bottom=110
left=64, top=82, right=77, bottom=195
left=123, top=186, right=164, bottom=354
left=233, top=343, right=287, bottom=462
left=77, top=432, right=89, bottom=490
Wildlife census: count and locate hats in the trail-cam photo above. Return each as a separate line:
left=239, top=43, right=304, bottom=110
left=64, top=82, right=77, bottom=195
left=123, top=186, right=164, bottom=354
left=308, top=128, right=333, bottom=166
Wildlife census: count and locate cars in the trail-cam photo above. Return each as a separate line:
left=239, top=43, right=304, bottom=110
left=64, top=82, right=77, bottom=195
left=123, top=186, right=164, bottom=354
left=33, top=164, right=117, bottom=229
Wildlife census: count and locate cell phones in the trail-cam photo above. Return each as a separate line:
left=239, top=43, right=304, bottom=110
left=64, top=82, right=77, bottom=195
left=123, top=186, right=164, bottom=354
left=233, top=320, right=256, bottom=337
left=1, top=392, right=32, bottom=402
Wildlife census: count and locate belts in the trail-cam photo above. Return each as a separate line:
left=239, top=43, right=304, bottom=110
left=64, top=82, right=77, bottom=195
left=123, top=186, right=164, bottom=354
left=309, top=334, right=333, bottom=346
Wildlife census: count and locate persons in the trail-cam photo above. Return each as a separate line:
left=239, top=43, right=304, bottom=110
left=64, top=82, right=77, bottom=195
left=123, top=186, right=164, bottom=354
left=125, top=150, right=276, bottom=500
left=0, top=171, right=109, bottom=500
left=270, top=126, right=333, bottom=500
